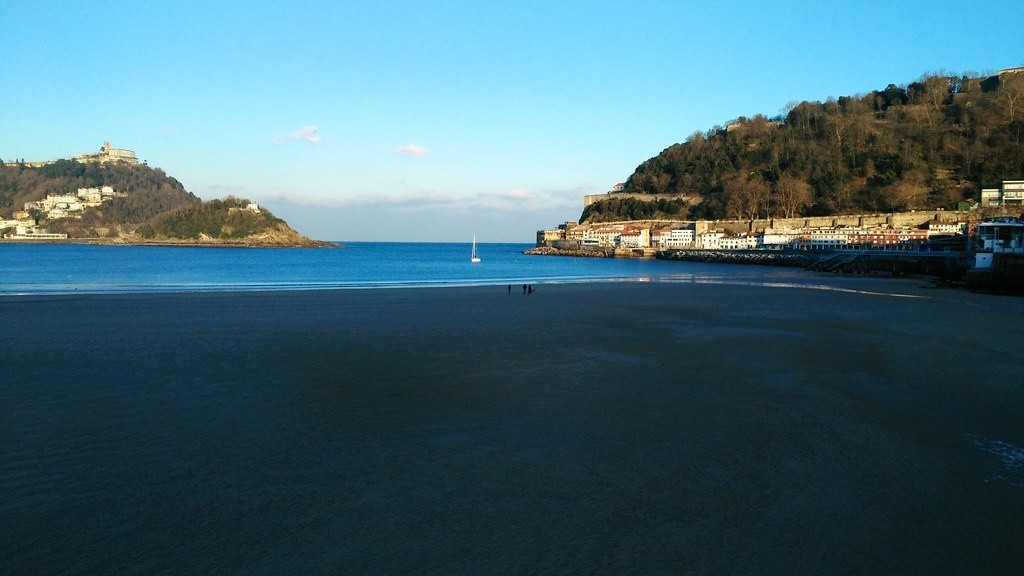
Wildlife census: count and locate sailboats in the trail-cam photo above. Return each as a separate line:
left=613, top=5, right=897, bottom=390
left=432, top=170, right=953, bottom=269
left=470, top=234, right=481, bottom=262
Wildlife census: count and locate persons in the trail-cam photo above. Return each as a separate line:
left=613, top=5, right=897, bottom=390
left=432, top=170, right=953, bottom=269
left=523, top=284, right=527, bottom=294
left=1010, top=237, right=1018, bottom=253
left=528, top=285, right=532, bottom=293
left=508, top=285, right=511, bottom=294
